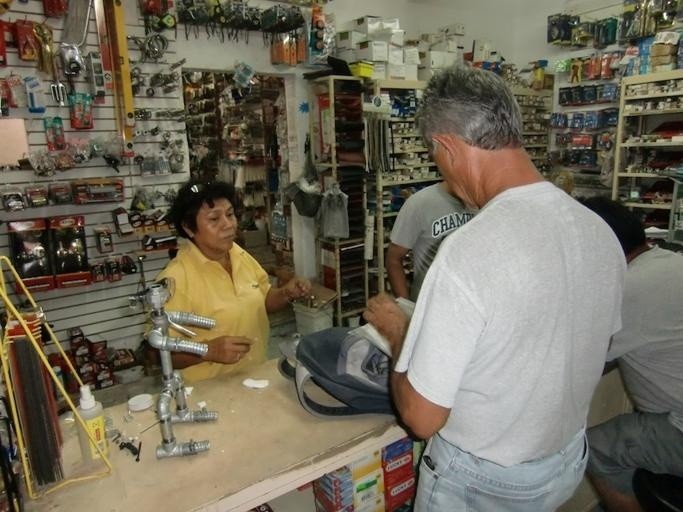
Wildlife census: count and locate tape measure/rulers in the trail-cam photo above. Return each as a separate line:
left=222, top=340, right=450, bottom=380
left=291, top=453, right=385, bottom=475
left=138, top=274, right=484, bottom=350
left=157, top=13, right=175, bottom=29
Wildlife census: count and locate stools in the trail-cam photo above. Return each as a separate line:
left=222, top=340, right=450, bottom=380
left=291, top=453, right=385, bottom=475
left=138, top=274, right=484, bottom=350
left=632, top=467, right=683, bottom=512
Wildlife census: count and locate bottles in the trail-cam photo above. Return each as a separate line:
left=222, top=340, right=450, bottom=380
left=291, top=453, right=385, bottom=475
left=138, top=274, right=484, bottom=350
left=270, top=209, right=286, bottom=237
left=74, top=385, right=110, bottom=475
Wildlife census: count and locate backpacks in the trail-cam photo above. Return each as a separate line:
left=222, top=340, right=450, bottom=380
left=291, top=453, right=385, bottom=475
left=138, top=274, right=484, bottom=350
left=278, top=324, right=399, bottom=414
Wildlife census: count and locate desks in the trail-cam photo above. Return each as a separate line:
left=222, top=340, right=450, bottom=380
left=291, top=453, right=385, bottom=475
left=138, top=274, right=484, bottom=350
left=19, top=356, right=635, bottom=512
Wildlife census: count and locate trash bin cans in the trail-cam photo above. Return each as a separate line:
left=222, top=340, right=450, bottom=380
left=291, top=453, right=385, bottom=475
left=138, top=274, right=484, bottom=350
left=293, top=297, right=335, bottom=337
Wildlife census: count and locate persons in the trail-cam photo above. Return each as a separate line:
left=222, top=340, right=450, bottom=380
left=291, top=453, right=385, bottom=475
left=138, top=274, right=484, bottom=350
left=360, top=64, right=629, bottom=512
left=575, top=192, right=681, bottom=512
left=382, top=179, right=481, bottom=304
left=145, top=179, right=314, bottom=374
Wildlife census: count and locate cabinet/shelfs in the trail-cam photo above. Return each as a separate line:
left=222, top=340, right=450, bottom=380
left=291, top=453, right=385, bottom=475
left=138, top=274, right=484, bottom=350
left=181, top=70, right=683, bottom=326
